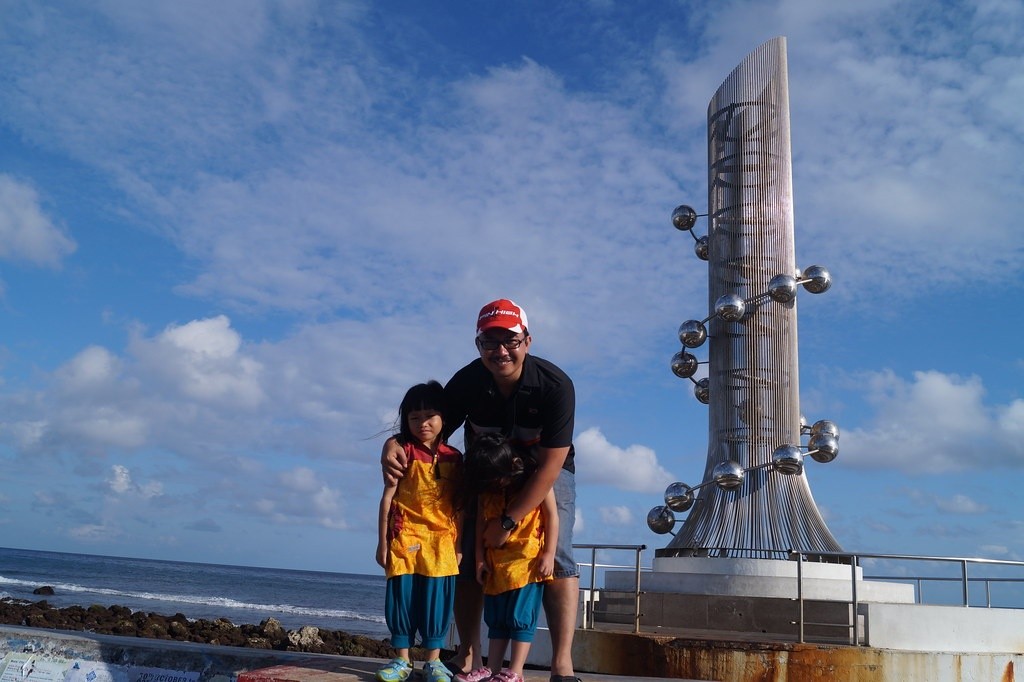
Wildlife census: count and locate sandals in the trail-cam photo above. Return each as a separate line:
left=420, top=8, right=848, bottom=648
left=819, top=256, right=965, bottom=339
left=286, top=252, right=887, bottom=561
left=423, top=659, right=453, bottom=682
left=453, top=668, right=491, bottom=682
left=488, top=669, right=526, bottom=682
left=378, top=659, right=414, bottom=682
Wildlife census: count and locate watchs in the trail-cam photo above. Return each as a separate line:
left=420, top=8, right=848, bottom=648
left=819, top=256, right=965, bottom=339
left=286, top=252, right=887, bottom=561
left=501, top=508, right=516, bottom=531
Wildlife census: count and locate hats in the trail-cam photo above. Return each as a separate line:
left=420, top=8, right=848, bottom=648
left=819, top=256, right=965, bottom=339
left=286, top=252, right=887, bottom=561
left=476, top=298, right=529, bottom=336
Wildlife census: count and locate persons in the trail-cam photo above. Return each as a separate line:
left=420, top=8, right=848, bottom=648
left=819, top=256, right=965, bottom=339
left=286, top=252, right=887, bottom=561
left=429, top=299, right=580, bottom=682
left=436, top=432, right=560, bottom=681
left=360, top=379, right=464, bottom=682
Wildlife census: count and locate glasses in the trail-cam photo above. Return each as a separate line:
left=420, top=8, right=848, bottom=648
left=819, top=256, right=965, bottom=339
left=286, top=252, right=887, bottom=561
left=477, top=337, right=527, bottom=349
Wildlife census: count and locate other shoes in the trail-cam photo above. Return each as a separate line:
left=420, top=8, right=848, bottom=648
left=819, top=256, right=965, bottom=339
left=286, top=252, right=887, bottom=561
left=548, top=674, right=580, bottom=682
